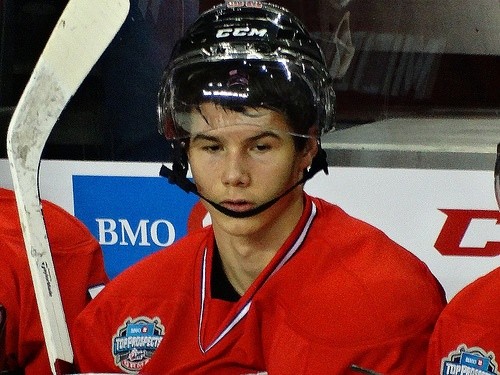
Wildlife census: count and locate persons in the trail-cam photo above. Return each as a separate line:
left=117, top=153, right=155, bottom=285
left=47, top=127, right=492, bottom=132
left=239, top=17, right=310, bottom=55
left=22, top=0, right=447, bottom=375
left=0, top=187, right=108, bottom=375
left=427, top=139, right=500, bottom=375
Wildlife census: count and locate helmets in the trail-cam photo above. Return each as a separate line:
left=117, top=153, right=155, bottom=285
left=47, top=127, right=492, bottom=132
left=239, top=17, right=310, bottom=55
left=159, top=0, right=338, bottom=141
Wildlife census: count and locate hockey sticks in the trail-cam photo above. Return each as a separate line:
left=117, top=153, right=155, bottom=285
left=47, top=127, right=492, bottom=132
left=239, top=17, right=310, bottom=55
left=7, top=0, right=131, bottom=375
